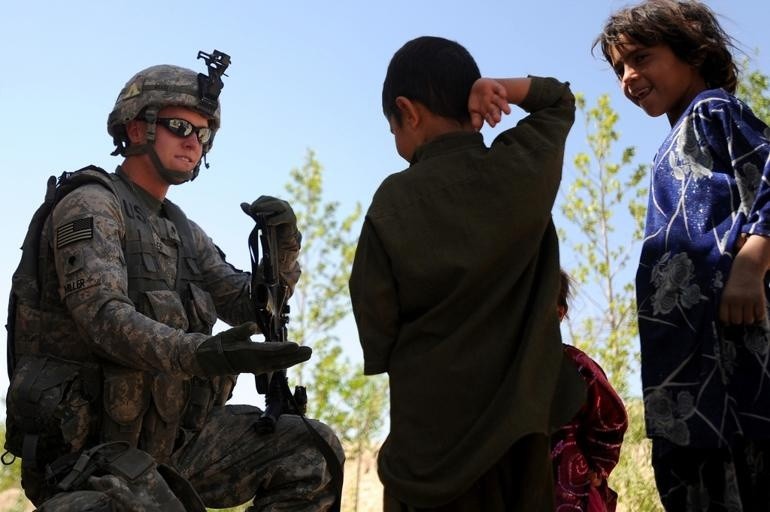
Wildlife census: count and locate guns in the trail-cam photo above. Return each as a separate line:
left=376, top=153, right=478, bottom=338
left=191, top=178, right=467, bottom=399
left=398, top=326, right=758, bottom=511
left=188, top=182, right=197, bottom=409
left=255, top=212, right=307, bottom=437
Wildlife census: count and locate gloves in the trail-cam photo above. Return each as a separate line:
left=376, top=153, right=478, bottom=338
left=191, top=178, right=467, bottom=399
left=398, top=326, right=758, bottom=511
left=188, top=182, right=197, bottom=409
left=240, top=195, right=301, bottom=273
left=195, top=323, right=312, bottom=378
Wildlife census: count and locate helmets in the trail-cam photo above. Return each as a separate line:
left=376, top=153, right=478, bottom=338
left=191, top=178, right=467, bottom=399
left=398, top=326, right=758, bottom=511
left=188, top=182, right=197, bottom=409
left=108, top=65, right=222, bottom=155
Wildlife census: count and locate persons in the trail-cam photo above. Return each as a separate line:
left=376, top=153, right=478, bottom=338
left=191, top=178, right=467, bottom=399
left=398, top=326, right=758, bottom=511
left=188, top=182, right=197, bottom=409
left=6, top=49, right=346, bottom=512
left=550, top=270, right=628, bottom=511
left=350, top=33, right=577, bottom=511
left=591, top=0, right=770, bottom=512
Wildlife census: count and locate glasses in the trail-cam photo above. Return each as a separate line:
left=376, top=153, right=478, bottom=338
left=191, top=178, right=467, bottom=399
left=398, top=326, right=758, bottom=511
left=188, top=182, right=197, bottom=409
left=137, top=114, right=212, bottom=146
